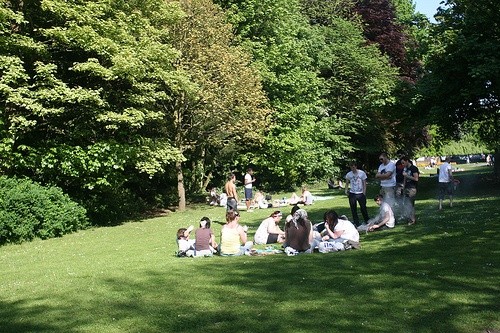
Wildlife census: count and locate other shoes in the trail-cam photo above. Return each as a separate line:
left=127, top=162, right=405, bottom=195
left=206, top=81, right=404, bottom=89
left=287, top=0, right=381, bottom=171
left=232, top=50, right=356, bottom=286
left=408, top=219, right=416, bottom=225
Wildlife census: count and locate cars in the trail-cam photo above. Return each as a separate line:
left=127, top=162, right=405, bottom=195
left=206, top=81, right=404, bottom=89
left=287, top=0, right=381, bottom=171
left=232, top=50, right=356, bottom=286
left=450, top=153, right=482, bottom=163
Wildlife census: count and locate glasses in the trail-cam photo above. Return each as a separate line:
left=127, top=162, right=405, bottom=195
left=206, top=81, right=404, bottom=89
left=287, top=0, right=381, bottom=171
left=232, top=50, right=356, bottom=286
left=397, top=153, right=402, bottom=157
left=379, top=157, right=383, bottom=161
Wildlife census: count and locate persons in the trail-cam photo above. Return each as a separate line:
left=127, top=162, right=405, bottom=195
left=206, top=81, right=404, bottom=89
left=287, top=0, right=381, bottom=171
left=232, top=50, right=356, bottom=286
left=286, top=208, right=321, bottom=253
left=194, top=215, right=220, bottom=257
left=343, top=160, right=370, bottom=226
left=224, top=172, right=239, bottom=219
left=216, top=209, right=254, bottom=257
left=367, top=193, right=396, bottom=231
left=244, top=168, right=256, bottom=212
left=323, top=208, right=361, bottom=249
left=396, top=148, right=413, bottom=220
left=176, top=224, right=196, bottom=257
left=438, top=155, right=454, bottom=210
left=376, top=151, right=397, bottom=218
left=254, top=210, right=286, bottom=244
left=284, top=205, right=301, bottom=224
left=400, top=156, right=420, bottom=226
left=290, top=184, right=314, bottom=206
